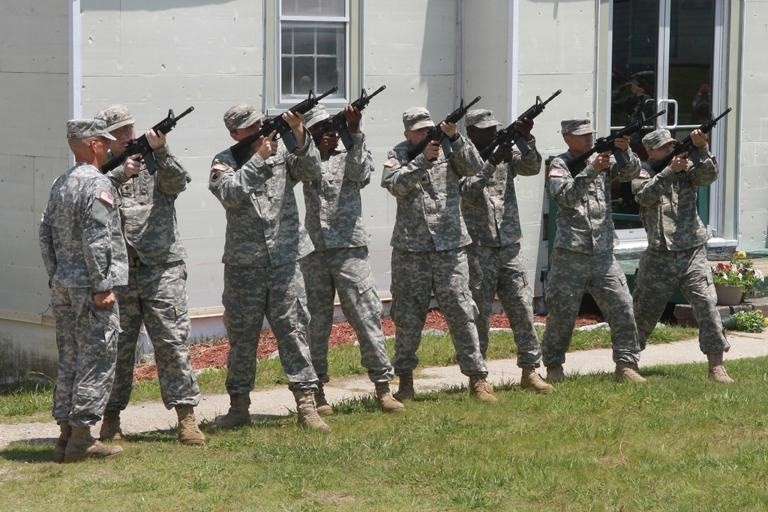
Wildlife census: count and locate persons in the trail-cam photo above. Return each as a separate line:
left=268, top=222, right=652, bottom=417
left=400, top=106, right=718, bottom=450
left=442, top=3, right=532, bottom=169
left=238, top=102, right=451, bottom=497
left=537, top=114, right=652, bottom=384
left=612, top=66, right=711, bottom=227
left=292, top=99, right=415, bottom=418
left=96, top=102, right=211, bottom=449
left=382, top=102, right=497, bottom=408
left=459, top=107, right=554, bottom=397
left=33, top=114, right=132, bottom=462
left=206, top=98, right=337, bottom=436
left=626, top=126, right=739, bottom=383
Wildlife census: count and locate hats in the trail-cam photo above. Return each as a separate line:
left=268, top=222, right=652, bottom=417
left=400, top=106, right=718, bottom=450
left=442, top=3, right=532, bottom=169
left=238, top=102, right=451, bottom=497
left=302, top=104, right=330, bottom=129
left=66, top=119, right=117, bottom=142
left=96, top=105, right=135, bottom=134
left=642, top=128, right=678, bottom=152
left=561, top=119, right=598, bottom=137
left=402, top=107, right=435, bottom=132
left=465, top=109, right=503, bottom=129
left=224, top=103, right=264, bottom=132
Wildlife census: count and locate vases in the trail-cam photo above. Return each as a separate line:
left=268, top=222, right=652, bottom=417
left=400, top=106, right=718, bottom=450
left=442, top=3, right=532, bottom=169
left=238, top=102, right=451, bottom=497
left=714, top=283, right=745, bottom=305
left=721, top=313, right=736, bottom=330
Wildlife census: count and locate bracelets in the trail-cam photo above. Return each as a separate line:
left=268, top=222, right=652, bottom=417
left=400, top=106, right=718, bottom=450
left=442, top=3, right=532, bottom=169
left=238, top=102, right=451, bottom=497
left=449, top=132, right=461, bottom=144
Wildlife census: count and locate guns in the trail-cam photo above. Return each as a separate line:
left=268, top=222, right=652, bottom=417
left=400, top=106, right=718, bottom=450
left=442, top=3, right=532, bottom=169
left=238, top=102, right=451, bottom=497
left=313, top=85, right=386, bottom=150
left=231, top=85, right=338, bottom=169
left=566, top=109, right=666, bottom=177
left=102, top=106, right=194, bottom=179
left=480, top=89, right=562, bottom=163
left=651, top=107, right=732, bottom=175
left=408, top=96, right=481, bottom=163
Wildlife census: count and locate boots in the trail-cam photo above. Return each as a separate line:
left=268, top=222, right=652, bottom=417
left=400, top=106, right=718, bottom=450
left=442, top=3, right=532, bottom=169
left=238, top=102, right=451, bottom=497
left=471, top=375, right=497, bottom=402
left=546, top=363, right=563, bottom=383
left=394, top=373, right=414, bottom=401
left=215, top=394, right=251, bottom=427
left=376, top=380, right=406, bottom=412
left=176, top=405, right=206, bottom=444
left=99, top=409, right=127, bottom=440
left=55, top=420, right=122, bottom=464
left=613, top=359, right=646, bottom=384
left=293, top=385, right=334, bottom=433
left=707, top=350, right=733, bottom=384
left=521, top=367, right=553, bottom=393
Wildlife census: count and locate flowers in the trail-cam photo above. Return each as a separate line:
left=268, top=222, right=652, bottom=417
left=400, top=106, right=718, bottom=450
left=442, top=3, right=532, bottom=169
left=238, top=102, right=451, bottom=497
left=732, top=309, right=768, bottom=333
left=711, top=251, right=764, bottom=297
